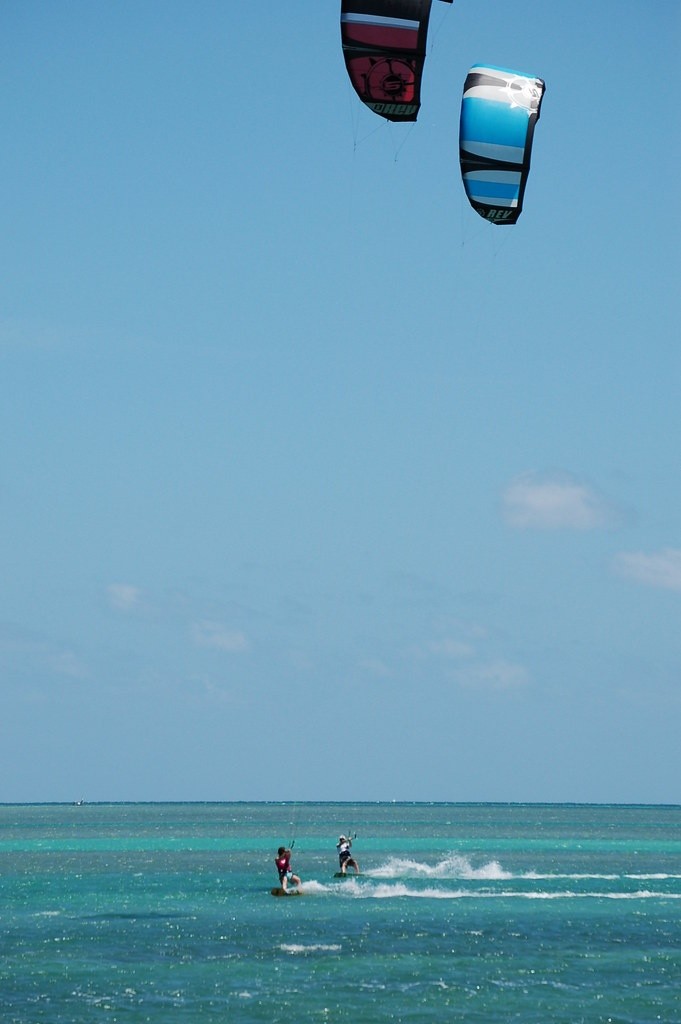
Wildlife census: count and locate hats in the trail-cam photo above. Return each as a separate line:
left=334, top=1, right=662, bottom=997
left=339, top=835, right=345, bottom=840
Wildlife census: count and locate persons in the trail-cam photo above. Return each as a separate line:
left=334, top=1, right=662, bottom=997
left=275, top=846, right=302, bottom=894
left=335, top=834, right=361, bottom=874
